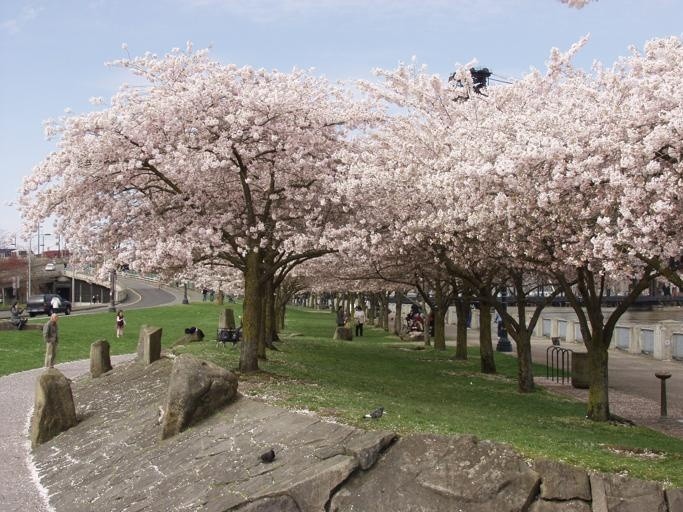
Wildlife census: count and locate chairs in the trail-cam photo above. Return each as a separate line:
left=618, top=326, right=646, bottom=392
left=215, top=323, right=242, bottom=347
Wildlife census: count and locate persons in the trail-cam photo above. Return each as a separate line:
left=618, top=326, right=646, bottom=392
left=201, top=287, right=209, bottom=302
left=115, top=309, right=126, bottom=335
left=43, top=312, right=59, bottom=370
left=404, top=304, right=435, bottom=336
left=9, top=301, right=22, bottom=329
left=50, top=295, right=60, bottom=314
left=184, top=325, right=206, bottom=342
left=352, top=305, right=366, bottom=336
left=492, top=309, right=509, bottom=341
left=335, top=305, right=345, bottom=327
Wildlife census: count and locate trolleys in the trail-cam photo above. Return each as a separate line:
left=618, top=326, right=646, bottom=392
left=216, top=326, right=242, bottom=348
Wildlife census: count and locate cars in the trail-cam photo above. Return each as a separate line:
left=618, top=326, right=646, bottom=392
left=45, top=263, right=55, bottom=270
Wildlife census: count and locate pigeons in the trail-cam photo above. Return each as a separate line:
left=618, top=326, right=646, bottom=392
left=257, top=448, right=275, bottom=463
left=362, top=407, right=384, bottom=418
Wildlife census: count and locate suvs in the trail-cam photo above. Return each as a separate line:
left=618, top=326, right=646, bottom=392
left=27, top=294, right=72, bottom=317
